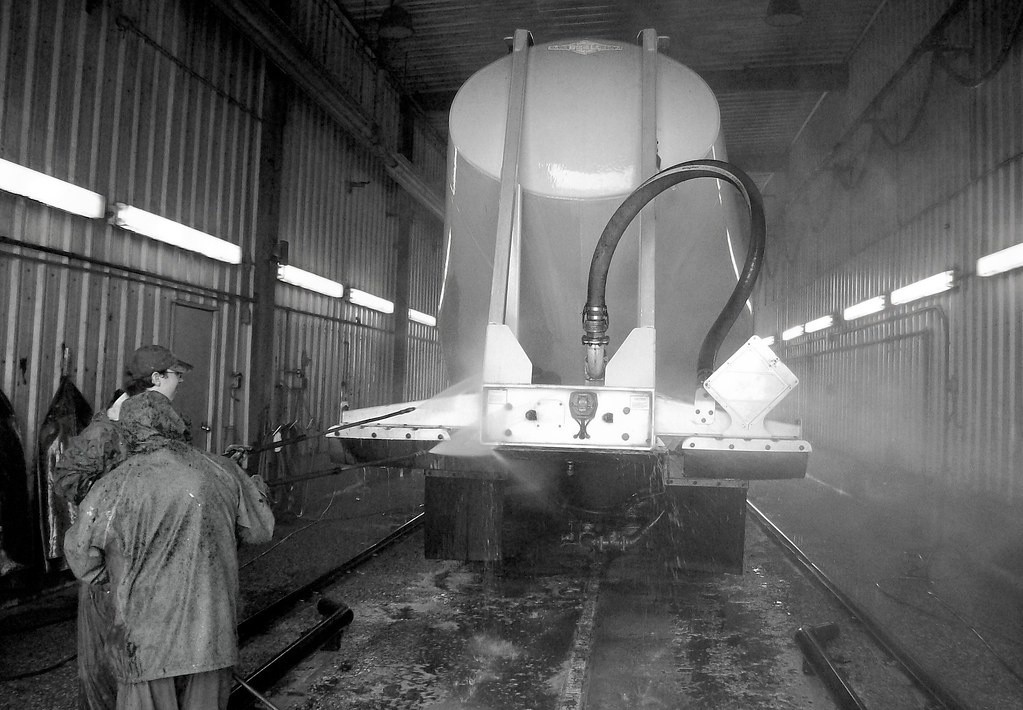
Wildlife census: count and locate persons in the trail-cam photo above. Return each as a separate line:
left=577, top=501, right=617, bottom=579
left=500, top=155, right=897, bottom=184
left=49, top=342, right=277, bottom=710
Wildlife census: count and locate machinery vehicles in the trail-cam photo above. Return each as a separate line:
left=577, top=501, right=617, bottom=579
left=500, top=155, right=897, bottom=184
left=324, top=22, right=813, bottom=577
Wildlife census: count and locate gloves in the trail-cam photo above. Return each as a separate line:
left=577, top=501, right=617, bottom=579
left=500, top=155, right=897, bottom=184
left=224, top=444, right=253, bottom=469
left=251, top=475, right=267, bottom=499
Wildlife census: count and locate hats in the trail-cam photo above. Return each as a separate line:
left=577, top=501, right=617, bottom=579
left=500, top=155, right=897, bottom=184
left=126, top=345, right=193, bottom=380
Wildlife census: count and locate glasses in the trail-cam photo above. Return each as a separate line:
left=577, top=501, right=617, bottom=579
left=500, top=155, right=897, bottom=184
left=165, top=371, right=183, bottom=379
